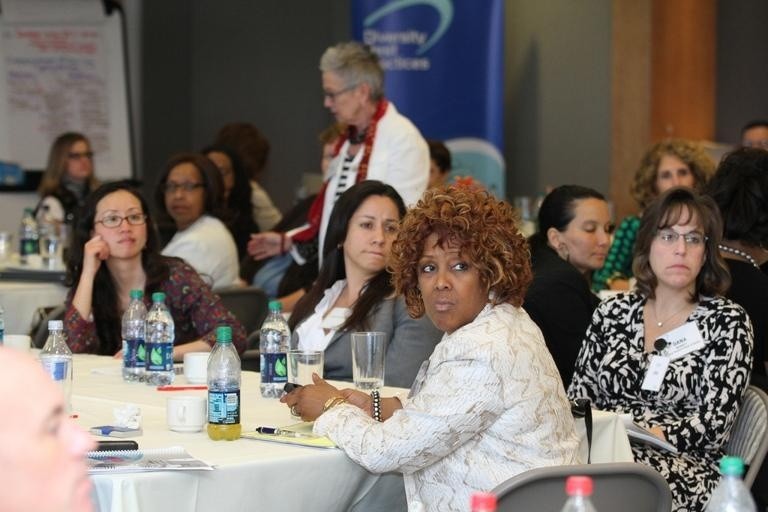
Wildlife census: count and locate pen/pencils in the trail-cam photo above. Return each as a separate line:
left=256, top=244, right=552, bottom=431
left=89, top=427, right=111, bottom=435
left=158, top=386, right=206, bottom=390
left=256, top=427, right=301, bottom=437
left=95, top=442, right=138, bottom=451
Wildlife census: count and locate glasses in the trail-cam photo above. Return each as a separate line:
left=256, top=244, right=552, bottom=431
left=324, top=83, right=360, bottom=100
left=94, top=212, right=146, bottom=229
left=163, top=180, right=205, bottom=192
left=654, top=229, right=710, bottom=248
left=68, top=151, right=93, bottom=159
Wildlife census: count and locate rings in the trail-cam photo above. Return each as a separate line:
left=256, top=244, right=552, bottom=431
left=289, top=404, right=302, bottom=417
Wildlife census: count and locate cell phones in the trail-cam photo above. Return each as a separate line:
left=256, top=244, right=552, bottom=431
left=96, top=440, right=139, bottom=452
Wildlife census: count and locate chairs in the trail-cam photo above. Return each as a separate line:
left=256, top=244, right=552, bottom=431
left=728, top=385, right=768, bottom=490
left=208, top=287, right=270, bottom=339
left=487, top=463, right=671, bottom=512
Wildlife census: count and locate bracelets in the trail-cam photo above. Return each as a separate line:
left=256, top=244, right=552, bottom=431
left=322, top=395, right=349, bottom=414
left=370, top=389, right=382, bottom=424
left=606, top=271, right=627, bottom=291
left=279, top=231, right=288, bottom=257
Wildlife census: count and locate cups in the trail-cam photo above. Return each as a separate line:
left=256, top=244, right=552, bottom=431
left=289, top=348, right=326, bottom=421
left=3, top=335, right=31, bottom=350
left=183, top=352, right=211, bottom=383
left=512, top=196, right=541, bottom=236
left=352, top=331, right=385, bottom=389
left=166, top=395, right=207, bottom=432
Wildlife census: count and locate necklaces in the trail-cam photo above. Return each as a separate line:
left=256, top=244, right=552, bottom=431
left=650, top=298, right=693, bottom=328
left=719, top=243, right=761, bottom=271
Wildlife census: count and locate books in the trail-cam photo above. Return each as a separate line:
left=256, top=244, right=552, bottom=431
left=86, top=446, right=218, bottom=476
left=571, top=404, right=679, bottom=456
left=240, top=422, right=340, bottom=449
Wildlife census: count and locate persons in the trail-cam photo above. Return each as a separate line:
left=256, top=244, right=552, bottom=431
left=735, top=114, right=767, bottom=151
left=152, top=150, right=240, bottom=292
left=285, top=178, right=447, bottom=391
left=279, top=174, right=584, bottom=512
left=424, top=137, right=454, bottom=190
left=565, top=185, right=755, bottom=511
left=219, top=119, right=284, bottom=234
left=591, top=135, right=720, bottom=293
left=240, top=122, right=350, bottom=315
left=0, top=343, right=102, bottom=512
left=244, top=40, right=433, bottom=293
left=61, top=179, right=248, bottom=366
left=700, top=144, right=768, bottom=395
left=517, top=183, right=610, bottom=392
left=159, top=141, right=262, bottom=288
left=36, top=131, right=109, bottom=231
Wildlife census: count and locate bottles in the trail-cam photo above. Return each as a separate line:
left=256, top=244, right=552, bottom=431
left=207, top=327, right=242, bottom=440
left=122, top=290, right=147, bottom=383
left=38, top=206, right=56, bottom=267
left=704, top=457, right=757, bottom=511
left=145, top=292, right=175, bottom=386
left=468, top=492, right=499, bottom=511
left=560, top=476, right=598, bottom=512
left=18, top=207, right=40, bottom=264
left=0, top=301, right=5, bottom=347
left=40, top=319, right=73, bottom=392
left=259, top=300, right=292, bottom=398
